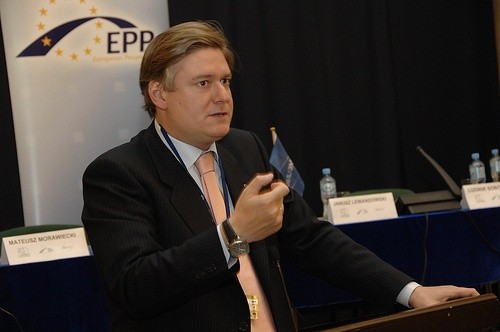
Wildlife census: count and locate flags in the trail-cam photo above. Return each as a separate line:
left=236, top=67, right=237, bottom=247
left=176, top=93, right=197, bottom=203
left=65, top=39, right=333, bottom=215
left=269, top=135, right=305, bottom=197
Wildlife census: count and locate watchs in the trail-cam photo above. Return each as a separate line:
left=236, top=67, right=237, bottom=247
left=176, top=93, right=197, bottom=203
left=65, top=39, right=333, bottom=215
left=222, top=219, right=249, bottom=257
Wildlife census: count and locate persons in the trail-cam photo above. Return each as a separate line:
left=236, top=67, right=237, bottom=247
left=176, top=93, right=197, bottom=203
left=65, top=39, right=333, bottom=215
left=81, top=22, right=481, bottom=332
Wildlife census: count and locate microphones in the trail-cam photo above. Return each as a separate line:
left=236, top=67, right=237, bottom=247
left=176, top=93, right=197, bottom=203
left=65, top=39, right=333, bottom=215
left=267, top=245, right=298, bottom=332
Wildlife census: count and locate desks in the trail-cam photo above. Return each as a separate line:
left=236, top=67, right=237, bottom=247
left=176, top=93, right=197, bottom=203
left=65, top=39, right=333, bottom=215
left=0, top=207, right=500, bottom=332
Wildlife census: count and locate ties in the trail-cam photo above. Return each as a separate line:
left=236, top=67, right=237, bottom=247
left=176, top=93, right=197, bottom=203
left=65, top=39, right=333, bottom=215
left=195, top=151, right=277, bottom=332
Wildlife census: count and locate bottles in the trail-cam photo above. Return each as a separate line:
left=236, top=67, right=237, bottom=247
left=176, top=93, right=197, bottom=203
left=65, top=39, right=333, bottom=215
left=489, top=149, right=500, bottom=181
left=469, top=153, right=486, bottom=184
left=320, top=168, right=337, bottom=221
